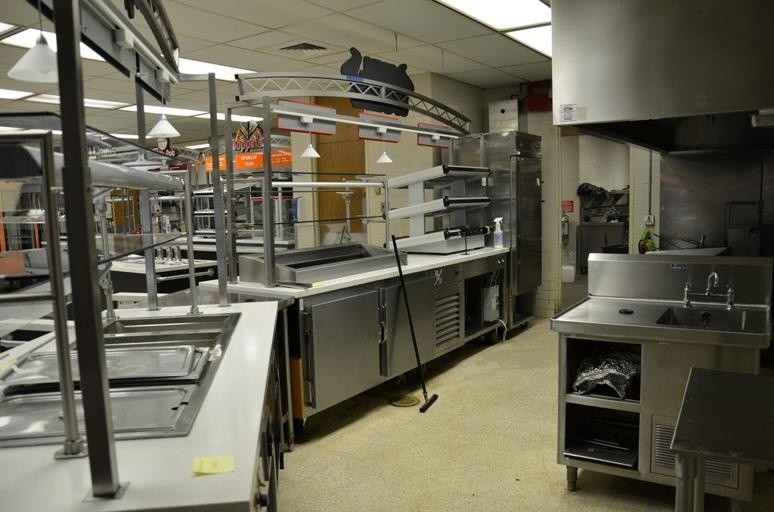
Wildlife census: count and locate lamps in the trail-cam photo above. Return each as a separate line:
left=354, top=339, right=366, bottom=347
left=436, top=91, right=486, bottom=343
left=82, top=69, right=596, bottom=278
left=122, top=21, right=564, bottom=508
left=6, top=0, right=57, bottom=83
left=299, top=124, right=321, bottom=158
left=374, top=134, right=392, bottom=164
left=144, top=80, right=181, bottom=138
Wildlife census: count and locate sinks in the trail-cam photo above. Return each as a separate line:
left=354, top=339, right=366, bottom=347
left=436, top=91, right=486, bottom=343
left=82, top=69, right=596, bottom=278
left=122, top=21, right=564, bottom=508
left=655, top=304, right=748, bottom=334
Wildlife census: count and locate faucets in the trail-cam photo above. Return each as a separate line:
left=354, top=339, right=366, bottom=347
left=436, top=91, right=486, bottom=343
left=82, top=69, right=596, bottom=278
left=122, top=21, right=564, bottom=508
left=683, top=272, right=736, bottom=311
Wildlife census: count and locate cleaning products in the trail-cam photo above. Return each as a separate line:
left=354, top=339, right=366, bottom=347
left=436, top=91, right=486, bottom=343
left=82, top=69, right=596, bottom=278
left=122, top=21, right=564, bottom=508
left=493, top=217, right=503, bottom=249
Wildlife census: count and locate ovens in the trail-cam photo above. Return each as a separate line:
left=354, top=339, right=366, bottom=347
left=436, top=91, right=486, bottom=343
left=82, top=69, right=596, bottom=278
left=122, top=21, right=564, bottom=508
left=449, top=130, right=546, bottom=341
